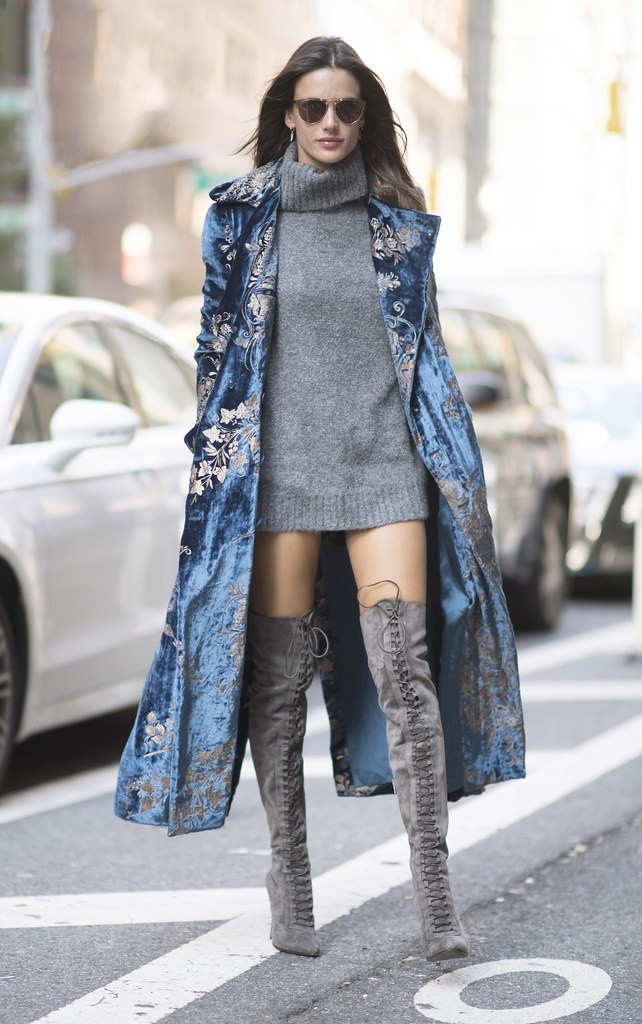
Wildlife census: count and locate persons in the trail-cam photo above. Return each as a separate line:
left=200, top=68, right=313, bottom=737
left=116, top=40, right=526, bottom=958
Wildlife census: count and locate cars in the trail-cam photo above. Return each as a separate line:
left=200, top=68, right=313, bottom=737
left=0, top=292, right=205, bottom=781
left=161, top=291, right=571, bottom=635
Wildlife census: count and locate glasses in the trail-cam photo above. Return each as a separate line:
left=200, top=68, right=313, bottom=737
left=290, top=98, right=367, bottom=126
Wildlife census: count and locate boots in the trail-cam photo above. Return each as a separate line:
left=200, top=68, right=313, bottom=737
left=354, top=580, right=470, bottom=960
left=244, top=612, right=329, bottom=959
